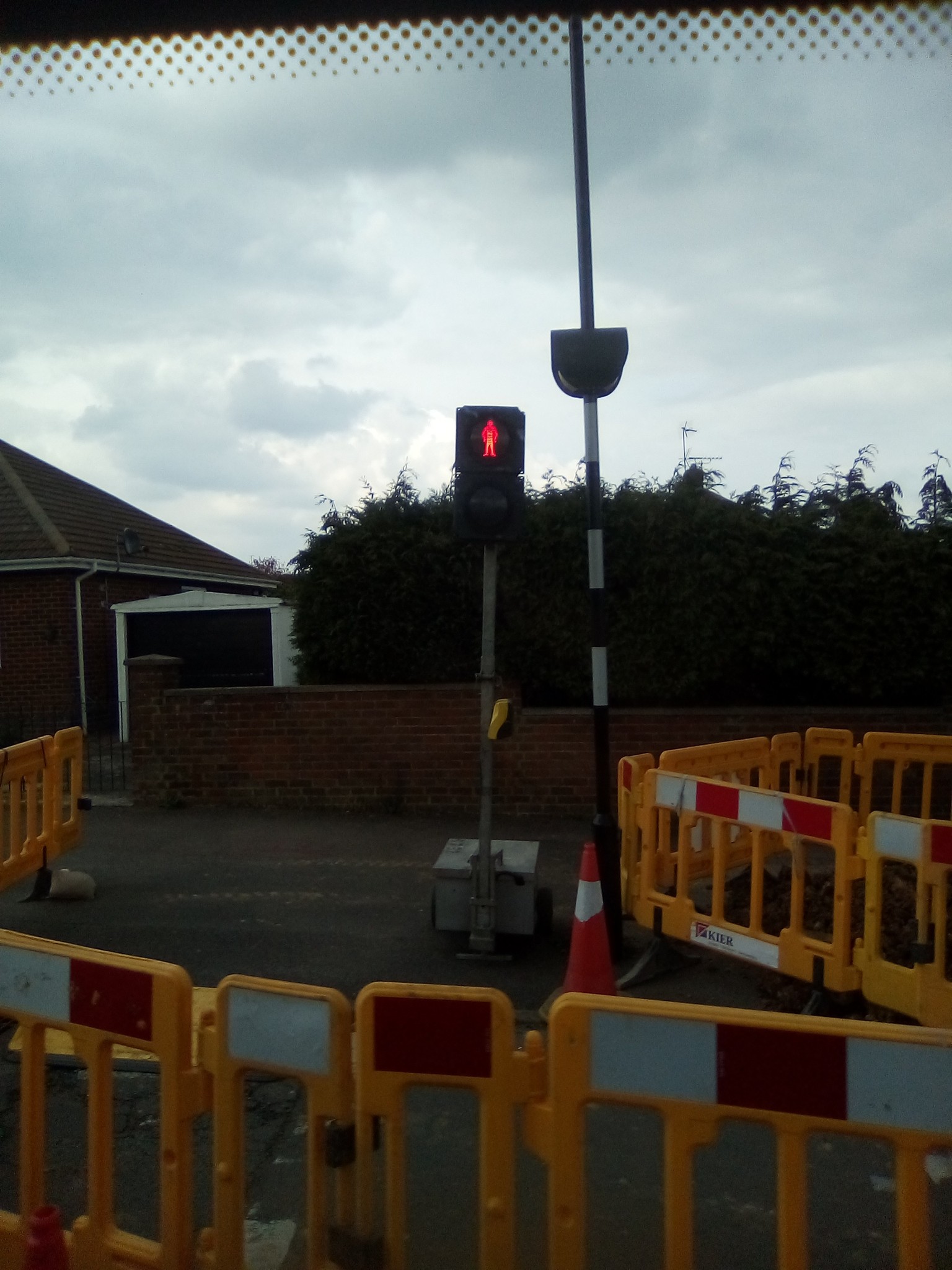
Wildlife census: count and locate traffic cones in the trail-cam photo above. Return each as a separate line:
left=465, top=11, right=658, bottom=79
left=536, top=842, right=623, bottom=1026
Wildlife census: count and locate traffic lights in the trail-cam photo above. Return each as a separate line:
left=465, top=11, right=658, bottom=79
left=452, top=405, right=528, bottom=547
